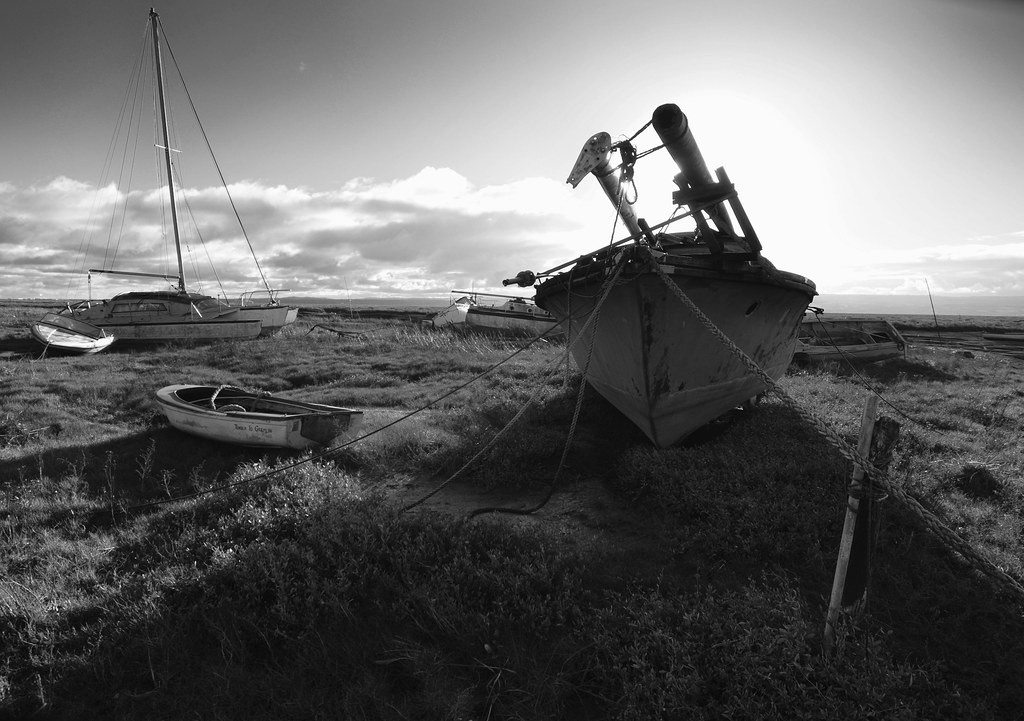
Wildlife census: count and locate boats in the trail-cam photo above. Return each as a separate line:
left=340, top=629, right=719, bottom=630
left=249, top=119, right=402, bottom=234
left=224, top=289, right=299, bottom=328
left=30, top=311, right=114, bottom=355
left=530, top=103, right=817, bottom=449
left=421, top=296, right=476, bottom=334
left=465, top=296, right=562, bottom=333
left=156, top=382, right=365, bottom=453
left=795, top=314, right=907, bottom=360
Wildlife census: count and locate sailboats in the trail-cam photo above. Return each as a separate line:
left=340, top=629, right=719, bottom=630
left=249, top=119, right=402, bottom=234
left=40, top=5, right=264, bottom=342
